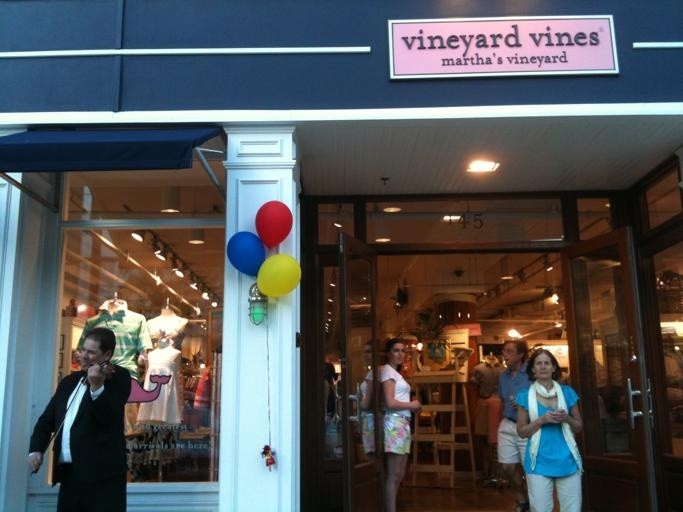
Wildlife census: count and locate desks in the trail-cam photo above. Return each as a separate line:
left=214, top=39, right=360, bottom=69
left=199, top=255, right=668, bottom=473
left=410, top=372, right=475, bottom=488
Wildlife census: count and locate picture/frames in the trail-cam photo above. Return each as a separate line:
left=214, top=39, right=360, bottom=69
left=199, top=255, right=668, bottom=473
left=421, top=337, right=452, bottom=371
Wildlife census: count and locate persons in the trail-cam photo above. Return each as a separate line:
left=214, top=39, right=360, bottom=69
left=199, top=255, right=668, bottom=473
left=359, top=341, right=377, bottom=464
left=27, top=328, right=130, bottom=512
left=513, top=348, right=584, bottom=512
left=497, top=340, right=534, bottom=512
left=148, top=309, right=189, bottom=346
left=377, top=338, right=420, bottom=512
left=470, top=357, right=505, bottom=479
left=137, top=337, right=182, bottom=425
left=322, top=351, right=338, bottom=459
left=73, top=299, right=153, bottom=438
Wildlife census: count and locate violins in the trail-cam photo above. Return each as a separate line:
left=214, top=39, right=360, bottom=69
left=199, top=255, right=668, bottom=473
left=83, top=360, right=116, bottom=384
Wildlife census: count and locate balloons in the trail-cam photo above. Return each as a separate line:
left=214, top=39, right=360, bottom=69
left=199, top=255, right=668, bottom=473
left=227, top=231, right=265, bottom=275
left=257, top=254, right=302, bottom=298
left=256, top=201, right=293, bottom=251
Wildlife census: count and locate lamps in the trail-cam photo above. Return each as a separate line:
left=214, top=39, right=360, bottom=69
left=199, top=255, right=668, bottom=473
left=247, top=282, right=270, bottom=324
left=323, top=269, right=336, bottom=337
left=331, top=202, right=345, bottom=231
left=122, top=171, right=220, bottom=307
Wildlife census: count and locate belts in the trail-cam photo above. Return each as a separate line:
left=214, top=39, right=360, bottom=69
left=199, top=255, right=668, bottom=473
left=508, top=417, right=516, bottom=424
left=385, top=410, right=411, bottom=420
